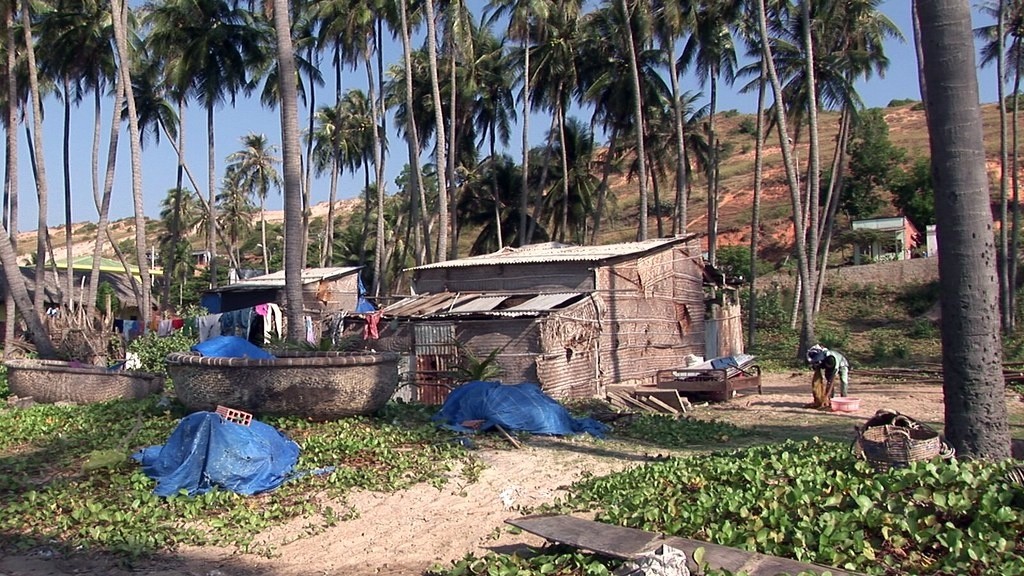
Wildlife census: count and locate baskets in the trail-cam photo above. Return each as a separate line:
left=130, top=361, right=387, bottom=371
left=854, top=408, right=956, bottom=473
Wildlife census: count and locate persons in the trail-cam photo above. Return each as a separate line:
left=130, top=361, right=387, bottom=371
left=812, top=350, right=848, bottom=406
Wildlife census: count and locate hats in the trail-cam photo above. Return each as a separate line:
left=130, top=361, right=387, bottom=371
left=806, top=344, right=825, bottom=364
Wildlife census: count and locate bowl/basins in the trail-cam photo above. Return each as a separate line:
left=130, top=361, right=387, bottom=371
left=830, top=396, right=861, bottom=411
